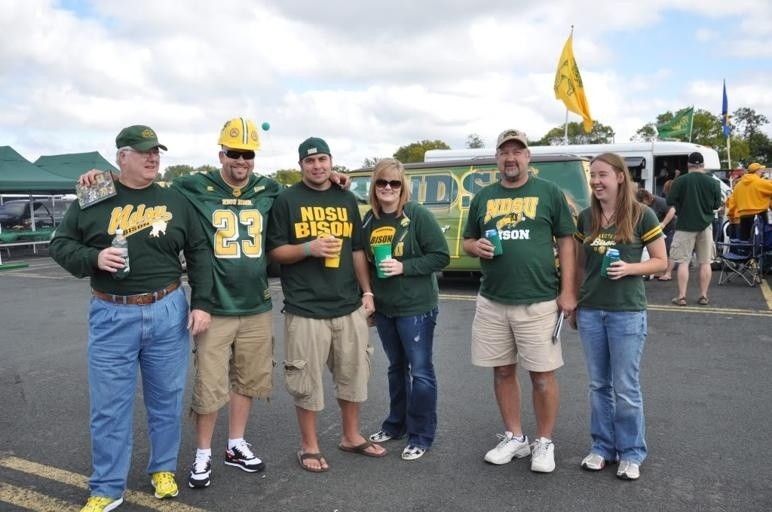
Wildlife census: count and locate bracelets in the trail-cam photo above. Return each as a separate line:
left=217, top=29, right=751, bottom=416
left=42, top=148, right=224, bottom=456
left=362, top=291, right=375, bottom=298
left=302, top=237, right=313, bottom=259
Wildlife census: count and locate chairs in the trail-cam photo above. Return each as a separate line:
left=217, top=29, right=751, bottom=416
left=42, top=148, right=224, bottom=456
left=716, top=216, right=765, bottom=287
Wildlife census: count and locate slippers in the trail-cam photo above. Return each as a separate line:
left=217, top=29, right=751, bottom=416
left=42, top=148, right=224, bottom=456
left=339, top=434, right=385, bottom=457
left=298, top=447, right=328, bottom=472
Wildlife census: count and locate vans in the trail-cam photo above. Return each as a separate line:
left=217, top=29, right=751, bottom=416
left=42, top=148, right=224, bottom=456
left=0, top=199, right=73, bottom=227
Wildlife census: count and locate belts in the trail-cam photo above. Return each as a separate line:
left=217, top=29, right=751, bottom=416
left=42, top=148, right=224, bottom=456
left=93, top=281, right=179, bottom=305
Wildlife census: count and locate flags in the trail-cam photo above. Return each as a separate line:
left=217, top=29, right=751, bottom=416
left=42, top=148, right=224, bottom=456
left=718, top=76, right=737, bottom=142
left=655, top=106, right=695, bottom=147
left=552, top=24, right=592, bottom=147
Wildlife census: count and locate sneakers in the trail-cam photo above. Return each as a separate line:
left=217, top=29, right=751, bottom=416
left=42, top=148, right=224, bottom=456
left=581, top=453, right=605, bottom=472
left=529, top=437, right=555, bottom=473
left=78, top=496, right=124, bottom=512
left=369, top=431, right=391, bottom=443
left=189, top=449, right=212, bottom=488
left=150, top=471, right=179, bottom=498
left=485, top=431, right=530, bottom=465
left=401, top=442, right=425, bottom=460
left=224, top=440, right=265, bottom=472
left=616, top=459, right=640, bottom=480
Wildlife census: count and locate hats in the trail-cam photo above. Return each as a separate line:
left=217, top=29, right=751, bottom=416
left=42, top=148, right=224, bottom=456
left=496, top=129, right=528, bottom=149
left=747, top=163, right=765, bottom=172
left=689, top=152, right=703, bottom=163
left=299, top=138, right=331, bottom=161
left=115, top=125, right=167, bottom=152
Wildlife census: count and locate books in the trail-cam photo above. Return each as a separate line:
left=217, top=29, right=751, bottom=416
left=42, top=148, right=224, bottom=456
left=76, top=169, right=119, bottom=214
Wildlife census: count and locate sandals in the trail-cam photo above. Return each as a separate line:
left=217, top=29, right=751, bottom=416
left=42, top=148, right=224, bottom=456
left=672, top=296, right=687, bottom=305
left=699, top=295, right=712, bottom=304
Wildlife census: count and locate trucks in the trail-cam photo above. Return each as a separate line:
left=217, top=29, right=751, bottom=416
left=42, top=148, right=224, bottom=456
left=424, top=140, right=735, bottom=281
left=337, top=152, right=599, bottom=273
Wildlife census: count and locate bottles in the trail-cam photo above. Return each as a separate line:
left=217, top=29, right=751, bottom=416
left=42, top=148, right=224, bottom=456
left=110, top=229, right=132, bottom=280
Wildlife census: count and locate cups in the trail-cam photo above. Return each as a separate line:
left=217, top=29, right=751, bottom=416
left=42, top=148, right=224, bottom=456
left=371, top=243, right=395, bottom=280
left=485, top=235, right=504, bottom=257
left=323, top=235, right=344, bottom=268
left=599, top=255, right=620, bottom=278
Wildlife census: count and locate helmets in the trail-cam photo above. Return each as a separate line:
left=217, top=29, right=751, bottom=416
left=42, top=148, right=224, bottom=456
left=224, top=150, right=255, bottom=159
left=217, top=118, right=260, bottom=151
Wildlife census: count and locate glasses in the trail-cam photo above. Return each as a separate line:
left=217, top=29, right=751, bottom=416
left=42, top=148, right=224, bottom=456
left=376, top=179, right=401, bottom=188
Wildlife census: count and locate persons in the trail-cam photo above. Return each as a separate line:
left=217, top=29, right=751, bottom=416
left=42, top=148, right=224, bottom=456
left=78, top=117, right=353, bottom=490
left=461, top=128, right=577, bottom=474
left=263, top=134, right=387, bottom=472
left=634, top=154, right=772, bottom=309
left=48, top=123, right=216, bottom=512
left=362, top=156, right=450, bottom=460
left=577, top=152, right=669, bottom=482
